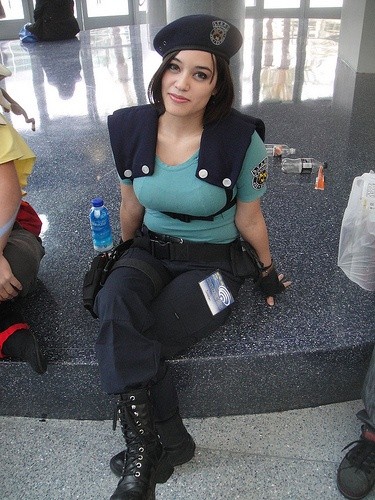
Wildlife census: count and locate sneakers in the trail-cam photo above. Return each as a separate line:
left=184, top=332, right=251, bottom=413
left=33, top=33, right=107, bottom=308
left=337, top=424, right=375, bottom=500
left=0, top=311, right=48, bottom=375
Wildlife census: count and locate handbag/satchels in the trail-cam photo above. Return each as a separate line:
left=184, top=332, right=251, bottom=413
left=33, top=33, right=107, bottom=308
left=83, top=251, right=113, bottom=319
left=62, top=14, right=80, bottom=40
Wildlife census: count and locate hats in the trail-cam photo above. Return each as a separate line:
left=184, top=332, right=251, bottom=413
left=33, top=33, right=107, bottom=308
left=154, top=14, right=243, bottom=62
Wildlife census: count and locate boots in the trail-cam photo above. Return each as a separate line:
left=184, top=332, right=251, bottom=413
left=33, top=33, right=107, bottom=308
left=110, top=392, right=195, bottom=476
left=109, top=389, right=174, bottom=500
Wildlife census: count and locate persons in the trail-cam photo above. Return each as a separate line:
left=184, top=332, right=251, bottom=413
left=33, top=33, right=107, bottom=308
left=0, top=62, right=47, bottom=374
left=337, top=344, right=375, bottom=500
left=24, top=0, right=80, bottom=42
left=93, top=14, right=293, bottom=500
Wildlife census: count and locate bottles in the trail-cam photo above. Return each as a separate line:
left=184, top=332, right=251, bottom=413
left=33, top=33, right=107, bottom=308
left=89, top=198, right=114, bottom=251
left=264, top=143, right=296, bottom=157
left=281, top=158, right=328, bottom=174
